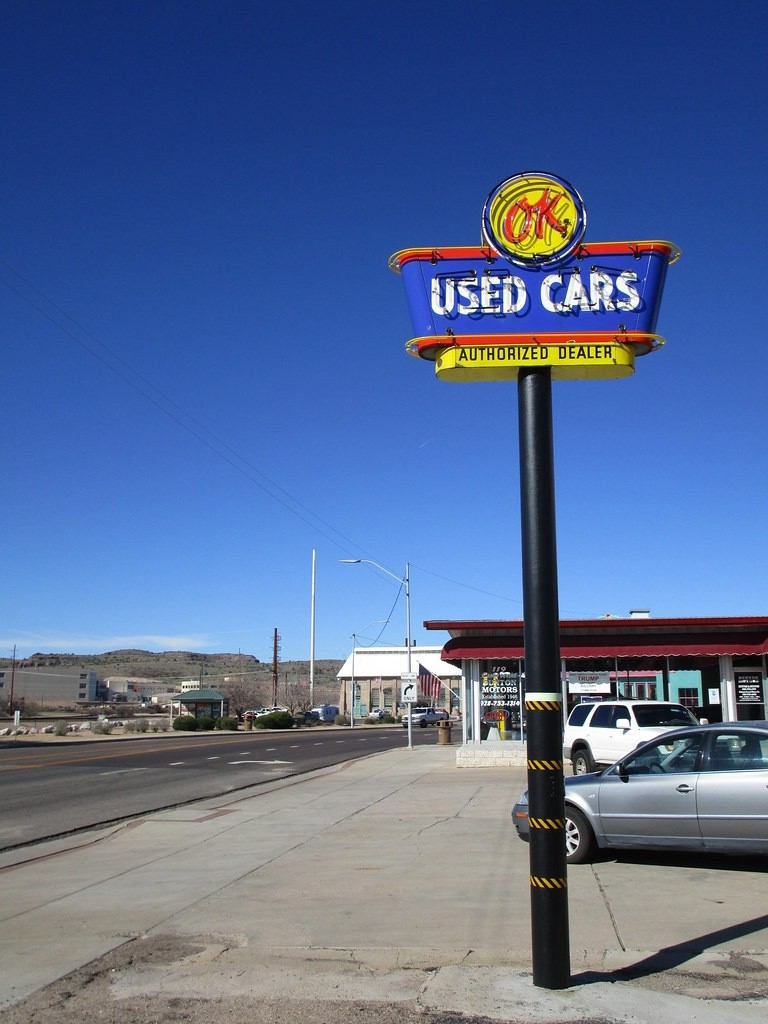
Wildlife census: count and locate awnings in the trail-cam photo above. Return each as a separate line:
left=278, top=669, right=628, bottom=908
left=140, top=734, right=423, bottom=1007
left=441, top=631, right=768, bottom=669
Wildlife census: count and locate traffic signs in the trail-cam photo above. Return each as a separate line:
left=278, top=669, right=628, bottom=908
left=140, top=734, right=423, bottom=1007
left=400, top=682, right=417, bottom=703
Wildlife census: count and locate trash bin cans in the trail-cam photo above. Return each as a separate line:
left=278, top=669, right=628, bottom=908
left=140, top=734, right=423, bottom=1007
left=243, top=714, right=256, bottom=731
left=435, top=720, right=454, bottom=745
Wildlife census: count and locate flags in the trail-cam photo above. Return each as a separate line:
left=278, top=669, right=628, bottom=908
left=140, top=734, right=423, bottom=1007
left=418, top=663, right=441, bottom=699
left=374, top=677, right=380, bottom=684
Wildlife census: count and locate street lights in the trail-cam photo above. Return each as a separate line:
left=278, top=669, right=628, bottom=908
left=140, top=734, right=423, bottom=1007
left=350, top=620, right=391, bottom=727
left=339, top=559, right=413, bottom=749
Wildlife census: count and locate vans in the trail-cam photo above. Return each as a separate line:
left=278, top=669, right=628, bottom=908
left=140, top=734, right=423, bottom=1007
left=310, top=704, right=339, bottom=722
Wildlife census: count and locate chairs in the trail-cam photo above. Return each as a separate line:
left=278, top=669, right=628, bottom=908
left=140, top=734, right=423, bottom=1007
left=714, top=743, right=735, bottom=770
left=740, top=746, right=761, bottom=763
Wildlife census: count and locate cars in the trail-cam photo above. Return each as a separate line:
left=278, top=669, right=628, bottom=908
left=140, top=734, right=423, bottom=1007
left=510, top=720, right=768, bottom=864
left=236, top=708, right=271, bottom=721
left=483, top=718, right=497, bottom=726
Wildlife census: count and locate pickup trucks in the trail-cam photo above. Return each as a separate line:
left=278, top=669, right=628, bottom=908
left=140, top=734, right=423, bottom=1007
left=270, top=707, right=289, bottom=713
left=368, top=708, right=390, bottom=719
left=400, top=707, right=448, bottom=729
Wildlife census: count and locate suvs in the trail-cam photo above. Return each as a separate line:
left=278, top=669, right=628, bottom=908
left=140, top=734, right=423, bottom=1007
left=290, top=711, right=318, bottom=728
left=562, top=701, right=741, bottom=776
